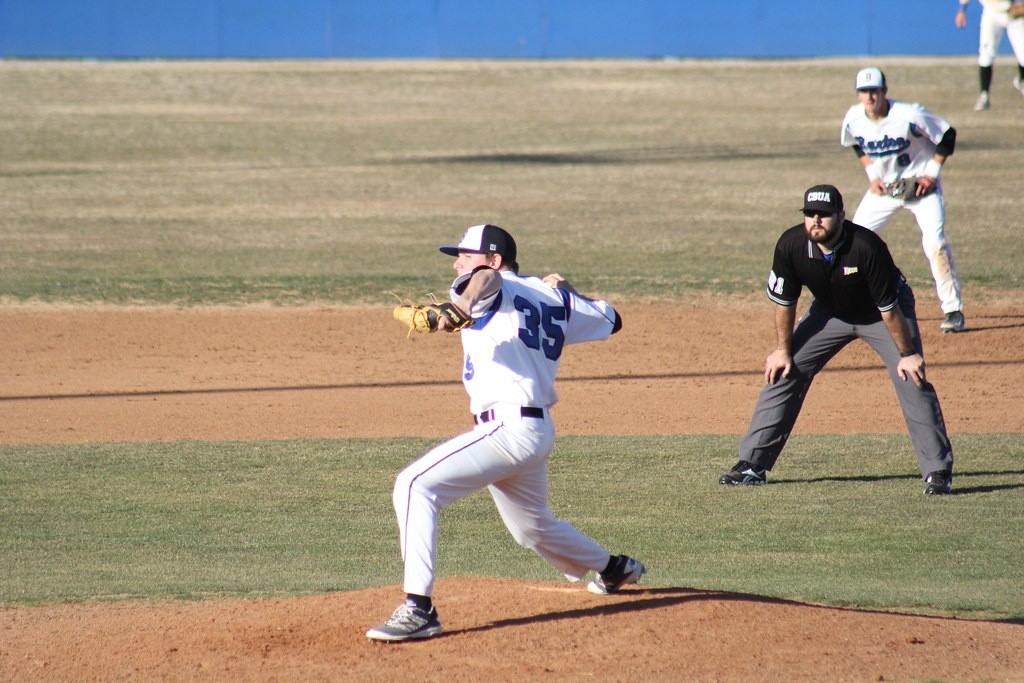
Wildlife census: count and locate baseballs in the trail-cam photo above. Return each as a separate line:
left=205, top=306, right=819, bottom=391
left=545, top=281, right=552, bottom=288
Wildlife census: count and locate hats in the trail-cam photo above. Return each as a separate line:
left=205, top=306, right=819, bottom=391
left=438, top=224, right=517, bottom=261
left=855, top=66, right=887, bottom=89
left=799, top=183, right=843, bottom=210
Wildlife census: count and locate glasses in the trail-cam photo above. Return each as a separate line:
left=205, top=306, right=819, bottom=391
left=803, top=210, right=831, bottom=218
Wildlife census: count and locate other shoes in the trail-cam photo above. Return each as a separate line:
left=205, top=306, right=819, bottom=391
left=1012, top=75, right=1024, bottom=95
left=974, top=98, right=990, bottom=111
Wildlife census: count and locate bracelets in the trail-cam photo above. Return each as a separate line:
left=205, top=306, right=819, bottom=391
left=900, top=348, right=917, bottom=357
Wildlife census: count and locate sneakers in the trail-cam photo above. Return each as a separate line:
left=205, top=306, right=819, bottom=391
left=365, top=599, right=443, bottom=641
left=940, top=310, right=964, bottom=332
left=922, top=470, right=952, bottom=495
left=719, top=460, right=766, bottom=485
left=587, top=555, right=644, bottom=594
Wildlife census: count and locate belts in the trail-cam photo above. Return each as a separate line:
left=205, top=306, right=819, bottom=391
left=474, top=407, right=543, bottom=424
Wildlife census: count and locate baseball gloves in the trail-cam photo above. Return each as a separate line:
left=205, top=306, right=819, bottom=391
left=1009, top=0, right=1024, bottom=17
left=390, top=291, right=476, bottom=339
left=887, top=177, right=937, bottom=203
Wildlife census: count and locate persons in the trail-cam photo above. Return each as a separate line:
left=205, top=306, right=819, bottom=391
left=839, top=67, right=963, bottom=333
left=955, top=0, right=1024, bottom=111
left=366, top=224, right=645, bottom=644
left=718, top=185, right=953, bottom=497
